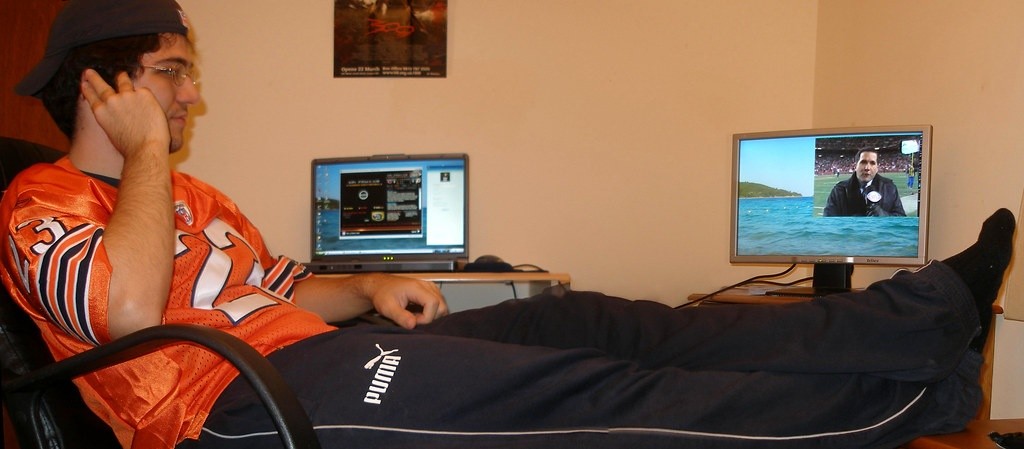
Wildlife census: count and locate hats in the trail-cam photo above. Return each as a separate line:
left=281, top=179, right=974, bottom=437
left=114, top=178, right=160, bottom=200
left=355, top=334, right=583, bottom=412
left=14, top=0, right=188, bottom=96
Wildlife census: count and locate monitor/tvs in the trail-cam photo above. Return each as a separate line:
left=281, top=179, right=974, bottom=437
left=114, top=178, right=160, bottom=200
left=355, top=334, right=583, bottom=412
left=729, top=124, right=933, bottom=296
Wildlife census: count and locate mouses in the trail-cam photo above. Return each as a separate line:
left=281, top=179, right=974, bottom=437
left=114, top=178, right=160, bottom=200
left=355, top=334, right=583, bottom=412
left=473, top=255, right=514, bottom=273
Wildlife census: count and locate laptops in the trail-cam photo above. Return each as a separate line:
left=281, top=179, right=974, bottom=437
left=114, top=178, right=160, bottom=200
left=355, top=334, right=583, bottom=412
left=297, top=153, right=469, bottom=270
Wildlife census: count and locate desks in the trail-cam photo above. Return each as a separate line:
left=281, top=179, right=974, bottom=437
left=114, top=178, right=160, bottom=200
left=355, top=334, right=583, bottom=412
left=316, top=271, right=572, bottom=295
left=689, top=288, right=1005, bottom=420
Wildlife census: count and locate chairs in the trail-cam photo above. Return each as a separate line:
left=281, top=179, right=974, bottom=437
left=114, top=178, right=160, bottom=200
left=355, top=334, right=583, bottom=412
left=0, top=137, right=322, bottom=449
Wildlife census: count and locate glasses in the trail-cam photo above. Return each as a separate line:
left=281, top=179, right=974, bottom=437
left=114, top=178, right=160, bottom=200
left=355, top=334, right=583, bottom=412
left=98, top=53, right=196, bottom=88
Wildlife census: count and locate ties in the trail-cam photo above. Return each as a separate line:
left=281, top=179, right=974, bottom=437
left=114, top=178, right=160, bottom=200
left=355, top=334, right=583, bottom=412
left=861, top=181, right=867, bottom=193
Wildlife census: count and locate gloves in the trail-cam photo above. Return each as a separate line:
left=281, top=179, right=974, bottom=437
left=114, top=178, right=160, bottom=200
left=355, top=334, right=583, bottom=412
left=866, top=202, right=889, bottom=217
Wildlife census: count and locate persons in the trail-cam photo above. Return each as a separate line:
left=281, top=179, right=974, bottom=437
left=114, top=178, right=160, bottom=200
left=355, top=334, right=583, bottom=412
left=0, top=1, right=1016, bottom=449
left=815, top=137, right=919, bottom=217
